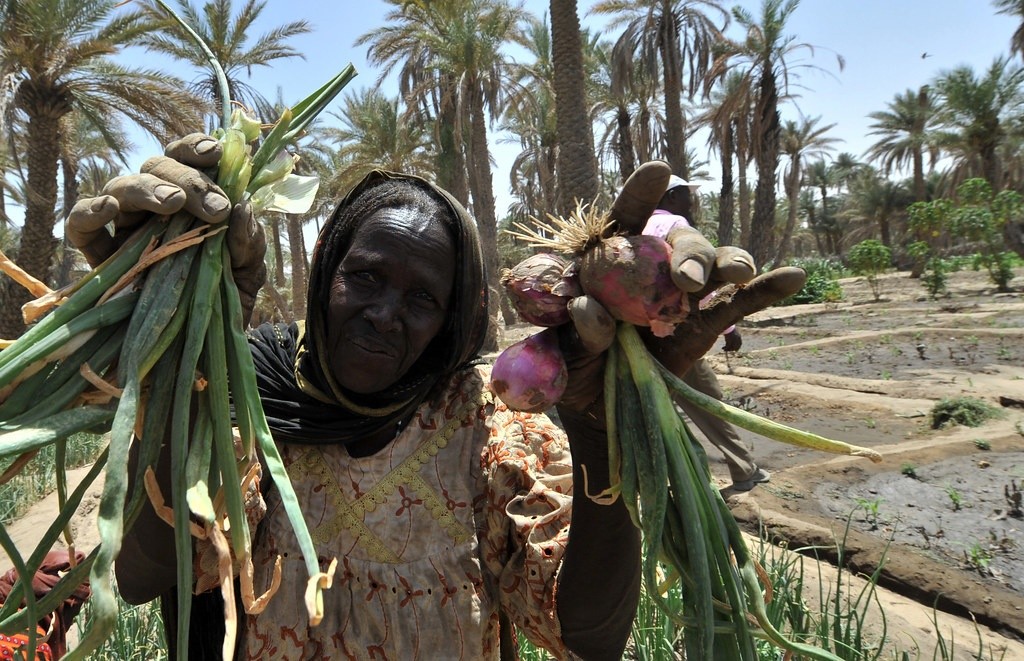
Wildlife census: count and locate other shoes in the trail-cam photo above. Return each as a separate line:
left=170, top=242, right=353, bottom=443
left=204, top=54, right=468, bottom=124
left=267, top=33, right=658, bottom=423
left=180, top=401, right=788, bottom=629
left=732, top=467, right=771, bottom=490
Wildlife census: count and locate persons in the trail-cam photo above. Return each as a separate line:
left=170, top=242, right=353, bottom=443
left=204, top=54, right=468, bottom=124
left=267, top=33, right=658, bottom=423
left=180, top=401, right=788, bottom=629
left=66, top=130, right=806, bottom=661
left=642, top=174, right=769, bottom=491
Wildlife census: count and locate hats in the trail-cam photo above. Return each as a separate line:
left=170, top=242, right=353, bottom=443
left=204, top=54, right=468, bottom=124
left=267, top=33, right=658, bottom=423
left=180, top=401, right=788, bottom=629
left=665, top=174, right=701, bottom=192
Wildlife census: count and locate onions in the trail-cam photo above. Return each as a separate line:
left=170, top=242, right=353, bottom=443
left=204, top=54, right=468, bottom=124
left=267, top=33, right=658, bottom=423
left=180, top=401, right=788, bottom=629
left=487, top=194, right=694, bottom=415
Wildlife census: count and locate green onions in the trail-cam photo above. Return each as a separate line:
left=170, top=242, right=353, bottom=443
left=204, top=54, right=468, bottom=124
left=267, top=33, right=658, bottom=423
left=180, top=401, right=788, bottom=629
left=0, top=0, right=360, bottom=661
